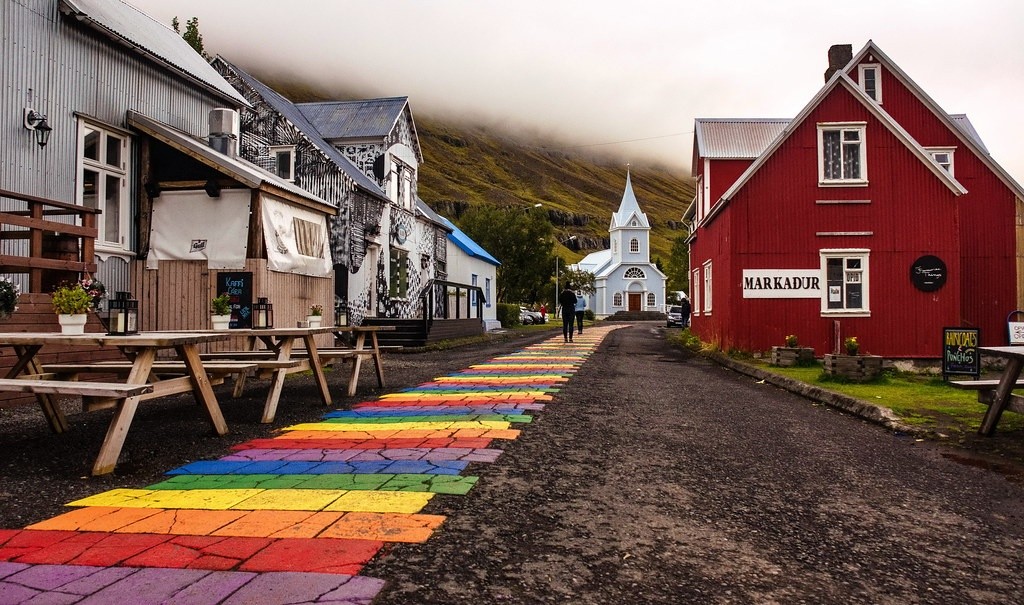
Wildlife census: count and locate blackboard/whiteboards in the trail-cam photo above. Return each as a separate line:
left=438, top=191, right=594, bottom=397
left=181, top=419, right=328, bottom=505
left=217, top=270, right=254, bottom=328
left=942, top=326, right=981, bottom=375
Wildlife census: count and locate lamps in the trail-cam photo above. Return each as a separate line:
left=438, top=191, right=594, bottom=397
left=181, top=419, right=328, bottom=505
left=372, top=219, right=382, bottom=236
left=23, top=107, right=53, bottom=150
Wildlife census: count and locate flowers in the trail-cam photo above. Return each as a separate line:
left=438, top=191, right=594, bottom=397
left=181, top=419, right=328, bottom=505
left=212, top=292, right=233, bottom=316
left=310, top=304, right=323, bottom=316
left=0, top=276, right=21, bottom=319
left=785, top=335, right=798, bottom=346
left=844, top=336, right=860, bottom=350
left=49, top=278, right=99, bottom=317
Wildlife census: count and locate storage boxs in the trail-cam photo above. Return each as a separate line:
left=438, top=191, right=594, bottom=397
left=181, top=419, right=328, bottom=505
left=297, top=320, right=308, bottom=328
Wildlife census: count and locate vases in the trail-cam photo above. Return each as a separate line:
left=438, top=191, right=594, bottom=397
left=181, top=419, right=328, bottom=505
left=789, top=345, right=795, bottom=348
left=58, top=313, right=87, bottom=335
left=846, top=347, right=858, bottom=356
left=211, top=315, right=231, bottom=329
left=307, top=315, right=323, bottom=328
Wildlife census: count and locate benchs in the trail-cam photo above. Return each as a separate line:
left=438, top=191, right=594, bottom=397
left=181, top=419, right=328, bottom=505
left=174, top=345, right=404, bottom=359
left=98, top=360, right=303, bottom=368
left=40, top=363, right=258, bottom=375
left=946, top=378, right=1024, bottom=393
left=0, top=376, right=155, bottom=399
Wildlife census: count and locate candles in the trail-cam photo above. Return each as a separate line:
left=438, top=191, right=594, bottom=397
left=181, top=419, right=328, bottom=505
left=340, top=315, right=346, bottom=325
left=258, top=312, right=266, bottom=327
left=118, top=313, right=130, bottom=332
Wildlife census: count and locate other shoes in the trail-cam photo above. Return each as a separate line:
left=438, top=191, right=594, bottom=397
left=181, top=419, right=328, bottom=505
left=569, top=339, right=573, bottom=342
left=564, top=339, right=568, bottom=342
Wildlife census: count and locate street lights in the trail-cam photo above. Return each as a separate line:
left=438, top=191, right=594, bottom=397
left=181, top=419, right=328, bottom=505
left=556, top=235, right=577, bottom=322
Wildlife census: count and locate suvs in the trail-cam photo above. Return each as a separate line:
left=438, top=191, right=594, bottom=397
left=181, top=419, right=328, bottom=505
left=665, top=306, right=690, bottom=327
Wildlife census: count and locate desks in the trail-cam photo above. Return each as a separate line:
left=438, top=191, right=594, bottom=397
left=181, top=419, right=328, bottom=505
left=977, top=346, right=1024, bottom=436
left=232, top=325, right=395, bottom=398
left=0, top=327, right=231, bottom=478
left=139, top=327, right=337, bottom=424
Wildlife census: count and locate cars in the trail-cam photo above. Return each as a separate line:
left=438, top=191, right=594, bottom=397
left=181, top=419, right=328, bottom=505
left=519, top=307, right=550, bottom=325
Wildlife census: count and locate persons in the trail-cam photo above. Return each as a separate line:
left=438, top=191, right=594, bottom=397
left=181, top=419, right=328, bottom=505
left=539, top=306, right=545, bottom=319
left=560, top=285, right=577, bottom=343
left=681, top=300, right=691, bottom=328
left=574, top=294, right=586, bottom=334
